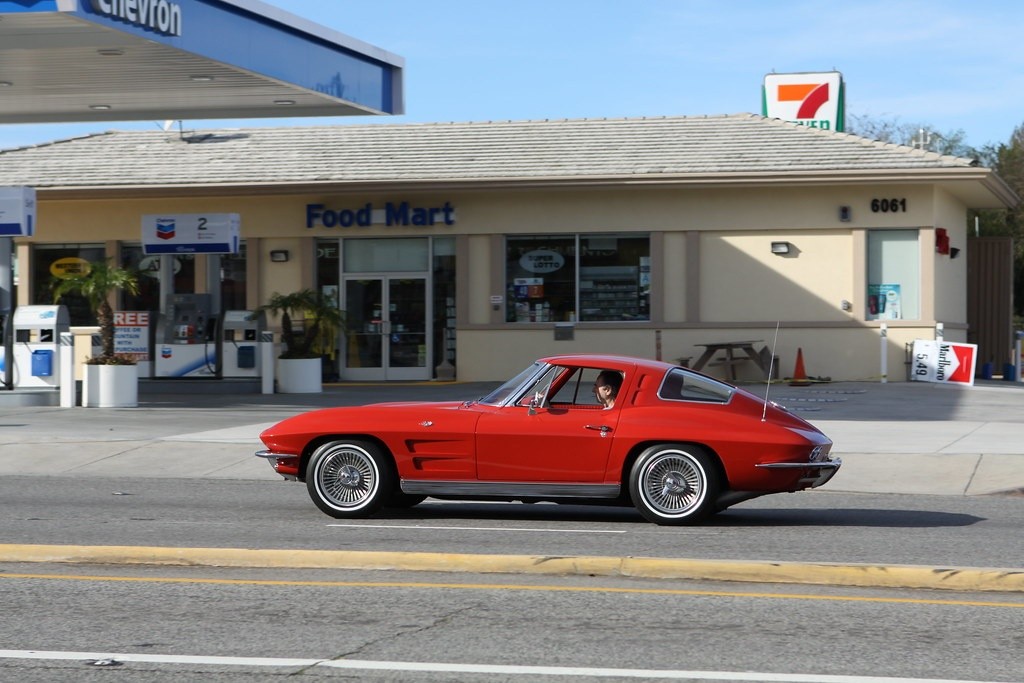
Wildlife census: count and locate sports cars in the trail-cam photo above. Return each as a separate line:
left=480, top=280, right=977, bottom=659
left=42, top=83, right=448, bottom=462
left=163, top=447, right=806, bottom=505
left=254, top=353, right=842, bottom=526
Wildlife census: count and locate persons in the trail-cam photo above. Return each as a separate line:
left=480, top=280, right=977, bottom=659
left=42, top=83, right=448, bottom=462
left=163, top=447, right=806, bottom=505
left=536, top=368, right=623, bottom=411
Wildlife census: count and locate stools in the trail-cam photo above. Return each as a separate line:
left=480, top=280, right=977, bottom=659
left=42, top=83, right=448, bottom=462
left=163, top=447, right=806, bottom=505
left=677, top=356, right=783, bottom=384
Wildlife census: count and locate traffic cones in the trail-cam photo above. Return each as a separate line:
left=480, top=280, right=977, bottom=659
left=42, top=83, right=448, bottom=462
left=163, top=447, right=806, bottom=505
left=784, top=348, right=811, bottom=387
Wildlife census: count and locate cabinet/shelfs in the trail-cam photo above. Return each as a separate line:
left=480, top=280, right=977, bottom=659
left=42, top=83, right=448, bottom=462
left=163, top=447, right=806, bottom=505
left=577, top=281, right=640, bottom=321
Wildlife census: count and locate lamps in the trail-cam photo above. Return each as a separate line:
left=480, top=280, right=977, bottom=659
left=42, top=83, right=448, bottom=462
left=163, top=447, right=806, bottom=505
left=269, top=249, right=289, bottom=263
left=771, top=241, right=790, bottom=254
left=951, top=246, right=961, bottom=261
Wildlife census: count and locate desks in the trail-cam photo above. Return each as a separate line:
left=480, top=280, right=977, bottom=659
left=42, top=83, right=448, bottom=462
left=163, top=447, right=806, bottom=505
left=691, top=340, right=771, bottom=384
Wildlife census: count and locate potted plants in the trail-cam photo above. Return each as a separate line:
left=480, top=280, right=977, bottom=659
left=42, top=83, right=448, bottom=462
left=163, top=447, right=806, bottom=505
left=51, top=258, right=142, bottom=408
left=244, top=287, right=351, bottom=394
